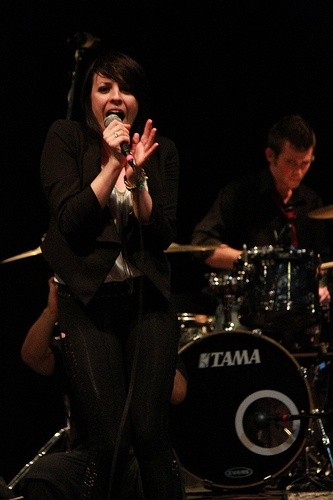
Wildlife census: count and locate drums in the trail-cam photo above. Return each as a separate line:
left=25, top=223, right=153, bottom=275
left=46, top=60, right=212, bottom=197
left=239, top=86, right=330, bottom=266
left=164, top=326, right=316, bottom=490
left=231, top=244, right=322, bottom=317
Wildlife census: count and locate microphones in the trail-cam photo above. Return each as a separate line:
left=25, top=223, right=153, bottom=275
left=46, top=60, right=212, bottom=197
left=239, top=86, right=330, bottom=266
left=104, top=114, right=135, bottom=168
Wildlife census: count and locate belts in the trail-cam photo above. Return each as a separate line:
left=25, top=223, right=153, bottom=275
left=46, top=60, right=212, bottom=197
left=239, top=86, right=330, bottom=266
left=55, top=274, right=155, bottom=299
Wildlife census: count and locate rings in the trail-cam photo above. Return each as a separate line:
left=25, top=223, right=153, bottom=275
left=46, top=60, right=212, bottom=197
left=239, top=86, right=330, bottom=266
left=114, top=132, right=119, bottom=137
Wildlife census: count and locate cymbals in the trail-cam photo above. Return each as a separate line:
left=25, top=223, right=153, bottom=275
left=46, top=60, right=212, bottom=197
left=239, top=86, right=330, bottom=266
left=162, top=242, right=219, bottom=255
left=309, top=201, right=333, bottom=221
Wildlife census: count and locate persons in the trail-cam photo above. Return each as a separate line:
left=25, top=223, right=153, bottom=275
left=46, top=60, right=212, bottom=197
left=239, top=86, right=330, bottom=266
left=21, top=277, right=188, bottom=500
left=41, top=38, right=186, bottom=500
left=191, top=116, right=331, bottom=303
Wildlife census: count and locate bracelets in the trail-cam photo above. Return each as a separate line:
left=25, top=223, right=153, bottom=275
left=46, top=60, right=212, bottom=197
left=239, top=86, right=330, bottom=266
left=124, top=168, right=147, bottom=192
